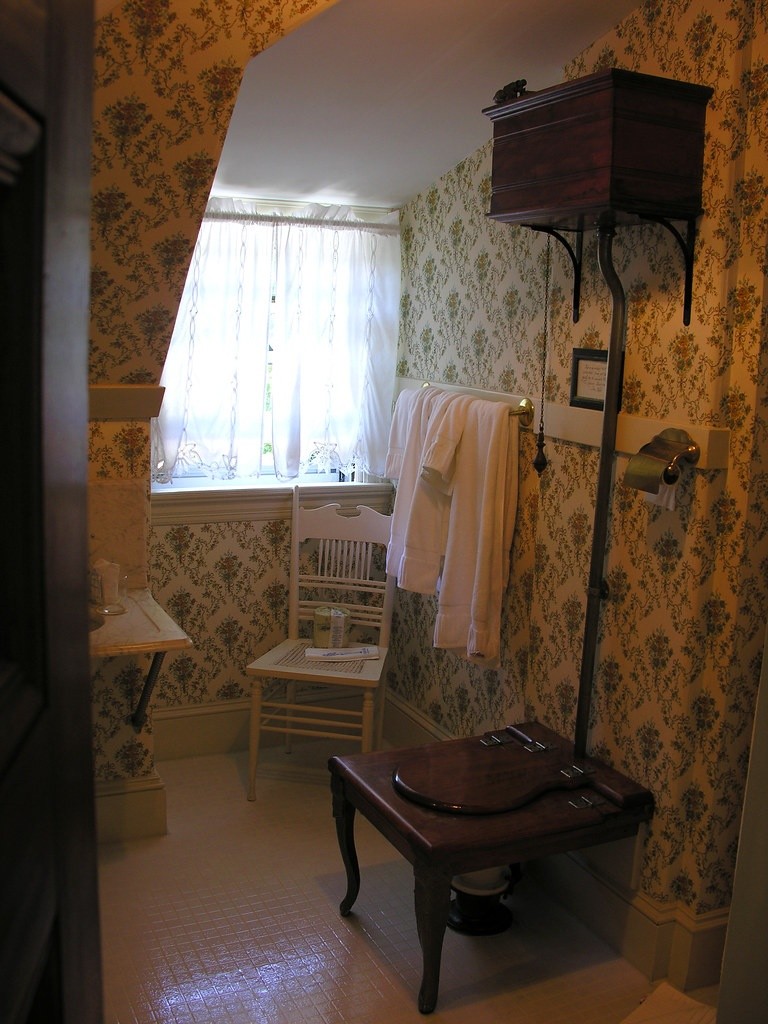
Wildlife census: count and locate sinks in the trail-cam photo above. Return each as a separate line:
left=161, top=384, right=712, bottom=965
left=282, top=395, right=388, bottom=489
left=86, top=608, right=104, bottom=633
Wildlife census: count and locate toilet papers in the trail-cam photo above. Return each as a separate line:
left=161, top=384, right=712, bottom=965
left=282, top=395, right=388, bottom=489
left=313, top=606, right=351, bottom=647
left=636, top=464, right=682, bottom=511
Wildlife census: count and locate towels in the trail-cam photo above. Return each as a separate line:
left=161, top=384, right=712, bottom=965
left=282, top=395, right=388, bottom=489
left=383, top=385, right=520, bottom=670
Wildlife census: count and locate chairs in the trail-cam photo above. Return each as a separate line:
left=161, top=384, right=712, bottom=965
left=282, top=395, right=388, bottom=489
left=244, top=486, right=395, bottom=802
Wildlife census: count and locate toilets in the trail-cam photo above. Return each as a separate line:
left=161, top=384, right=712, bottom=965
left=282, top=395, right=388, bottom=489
left=324, top=714, right=654, bottom=1016
